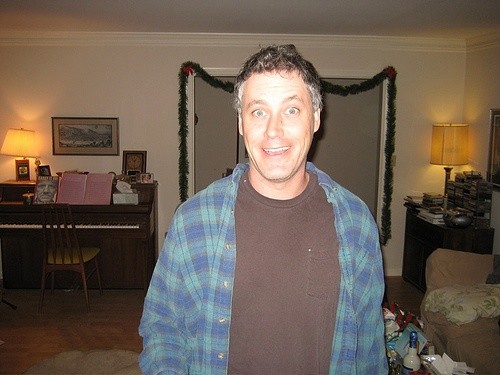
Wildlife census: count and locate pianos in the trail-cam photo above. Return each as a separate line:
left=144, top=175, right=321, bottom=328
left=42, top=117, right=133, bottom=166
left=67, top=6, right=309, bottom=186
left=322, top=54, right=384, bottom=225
left=0, top=180, right=158, bottom=290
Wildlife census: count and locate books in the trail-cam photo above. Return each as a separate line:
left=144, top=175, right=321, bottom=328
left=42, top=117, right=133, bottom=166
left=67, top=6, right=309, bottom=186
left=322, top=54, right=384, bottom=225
left=57, top=172, right=112, bottom=204
left=33, top=176, right=58, bottom=204
left=403, top=171, right=492, bottom=227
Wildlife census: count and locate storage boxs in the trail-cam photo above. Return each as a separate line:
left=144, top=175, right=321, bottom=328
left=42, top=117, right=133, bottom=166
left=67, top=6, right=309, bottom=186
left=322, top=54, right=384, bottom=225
left=393, top=322, right=432, bottom=359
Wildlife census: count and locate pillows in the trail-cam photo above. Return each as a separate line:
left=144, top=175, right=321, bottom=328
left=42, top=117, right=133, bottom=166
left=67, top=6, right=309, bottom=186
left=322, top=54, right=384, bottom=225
left=424, top=282, right=500, bottom=325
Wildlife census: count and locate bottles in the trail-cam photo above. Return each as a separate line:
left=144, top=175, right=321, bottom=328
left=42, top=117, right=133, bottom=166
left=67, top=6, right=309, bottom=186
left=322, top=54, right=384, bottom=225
left=401, top=331, right=422, bottom=375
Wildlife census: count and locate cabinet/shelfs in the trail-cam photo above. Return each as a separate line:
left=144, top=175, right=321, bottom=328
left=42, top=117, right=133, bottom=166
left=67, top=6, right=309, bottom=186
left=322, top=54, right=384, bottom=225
left=402, top=202, right=495, bottom=294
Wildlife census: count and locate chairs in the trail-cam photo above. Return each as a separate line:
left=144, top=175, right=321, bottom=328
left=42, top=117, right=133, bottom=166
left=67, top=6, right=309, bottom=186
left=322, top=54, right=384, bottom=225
left=38, top=203, right=104, bottom=313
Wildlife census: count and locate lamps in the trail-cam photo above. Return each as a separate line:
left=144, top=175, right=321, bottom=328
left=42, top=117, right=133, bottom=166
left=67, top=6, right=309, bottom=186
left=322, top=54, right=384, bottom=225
left=1, top=128, right=40, bottom=182
left=430, top=122, right=471, bottom=198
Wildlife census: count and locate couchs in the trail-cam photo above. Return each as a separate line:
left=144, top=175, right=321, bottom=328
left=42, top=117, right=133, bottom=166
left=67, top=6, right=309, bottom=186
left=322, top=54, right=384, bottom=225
left=419, top=248, right=500, bottom=375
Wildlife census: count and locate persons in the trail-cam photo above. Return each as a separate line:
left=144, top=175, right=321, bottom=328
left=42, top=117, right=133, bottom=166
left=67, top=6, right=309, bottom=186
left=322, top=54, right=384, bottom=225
left=138, top=44, right=389, bottom=375
left=143, top=175, right=151, bottom=182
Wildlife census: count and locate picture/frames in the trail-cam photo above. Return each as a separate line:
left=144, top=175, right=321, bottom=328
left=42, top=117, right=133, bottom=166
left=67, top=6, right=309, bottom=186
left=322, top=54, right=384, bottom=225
left=122, top=150, right=147, bottom=173
left=487, top=108, right=500, bottom=192
left=51, top=116, right=120, bottom=156
left=141, top=173, right=154, bottom=184
